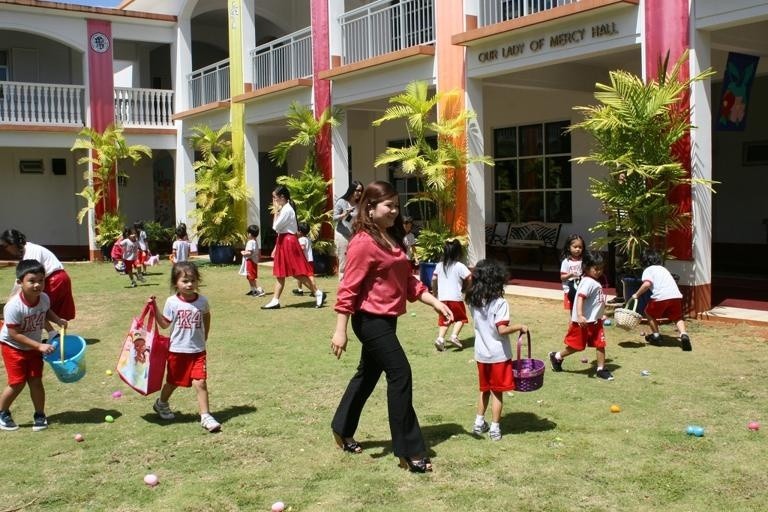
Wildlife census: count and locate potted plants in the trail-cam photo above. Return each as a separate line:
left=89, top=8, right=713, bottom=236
left=268, top=99, right=346, bottom=274
left=142, top=220, right=178, bottom=255
left=185, top=123, right=248, bottom=263
left=372, top=82, right=495, bottom=290
left=69, top=121, right=152, bottom=261
left=563, top=48, right=724, bottom=316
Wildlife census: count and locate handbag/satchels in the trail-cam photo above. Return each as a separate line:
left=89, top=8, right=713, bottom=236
left=117, top=316, right=170, bottom=396
left=238, top=256, right=248, bottom=277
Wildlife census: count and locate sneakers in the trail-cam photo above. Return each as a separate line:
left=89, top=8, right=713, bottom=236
left=473, top=420, right=489, bottom=434
left=681, top=334, right=692, bottom=351
left=0, top=409, right=19, bottom=431
left=137, top=276, right=146, bottom=282
left=434, top=338, right=446, bottom=352
left=292, top=289, right=303, bottom=296
left=549, top=351, right=564, bottom=372
left=153, top=398, right=175, bottom=420
left=201, top=414, right=220, bottom=433
left=645, top=333, right=664, bottom=346
left=32, top=411, right=48, bottom=432
left=595, top=367, right=613, bottom=380
left=130, top=280, right=137, bottom=287
left=246, top=290, right=265, bottom=297
left=449, top=336, right=463, bottom=348
left=487, top=427, right=502, bottom=440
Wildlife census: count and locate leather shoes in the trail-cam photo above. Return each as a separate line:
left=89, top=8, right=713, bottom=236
left=260, top=303, right=280, bottom=309
left=315, top=292, right=326, bottom=308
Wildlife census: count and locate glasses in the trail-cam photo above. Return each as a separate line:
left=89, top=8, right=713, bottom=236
left=355, top=188, right=363, bottom=193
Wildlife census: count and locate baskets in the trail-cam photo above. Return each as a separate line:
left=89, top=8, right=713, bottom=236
left=513, top=330, right=545, bottom=392
left=614, top=296, right=642, bottom=331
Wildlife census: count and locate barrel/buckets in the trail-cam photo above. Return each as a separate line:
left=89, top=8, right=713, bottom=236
left=43, top=324, right=86, bottom=384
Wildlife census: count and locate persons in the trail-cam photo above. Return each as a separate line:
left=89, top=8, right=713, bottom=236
left=0, top=229, right=76, bottom=342
left=331, top=181, right=453, bottom=474
left=0, top=259, right=69, bottom=431
left=240, top=179, right=421, bottom=311
left=549, top=234, right=692, bottom=381
left=464, top=258, right=528, bottom=443
left=111, top=220, right=191, bottom=287
left=430, top=238, right=473, bottom=351
left=149, top=260, right=223, bottom=434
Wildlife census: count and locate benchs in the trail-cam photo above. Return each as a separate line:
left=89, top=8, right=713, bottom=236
left=496, top=222, right=562, bottom=270
left=485, top=224, right=496, bottom=246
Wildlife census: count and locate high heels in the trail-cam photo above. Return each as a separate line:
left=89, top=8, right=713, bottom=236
left=332, top=428, right=363, bottom=453
left=396, top=454, right=432, bottom=472
